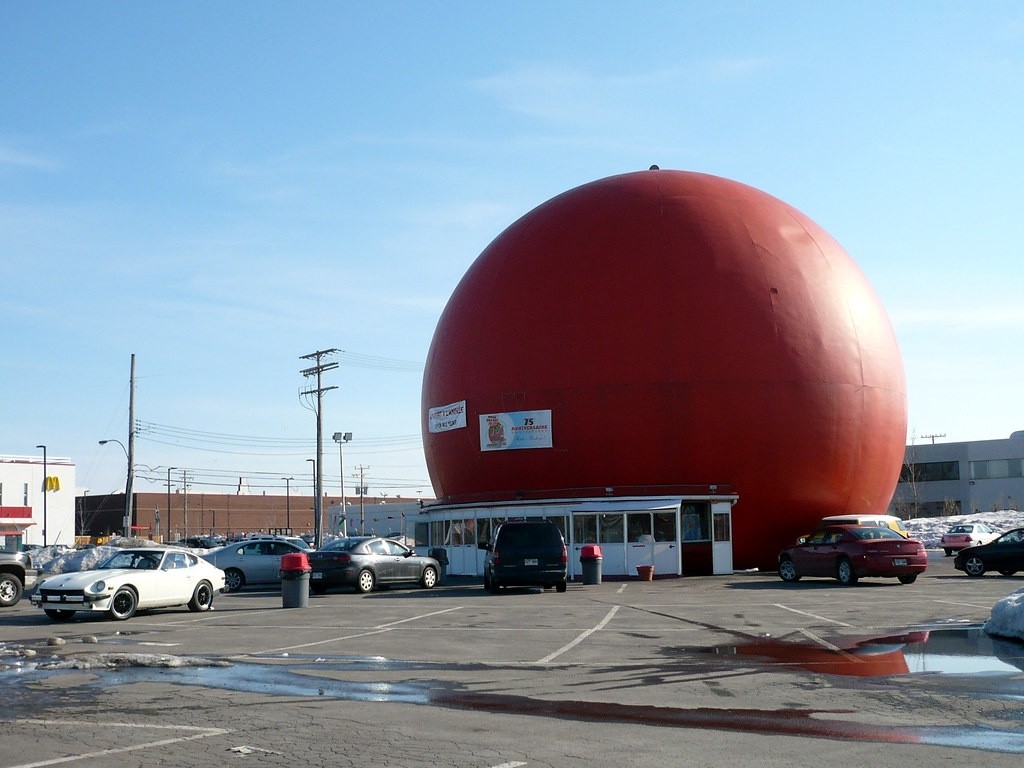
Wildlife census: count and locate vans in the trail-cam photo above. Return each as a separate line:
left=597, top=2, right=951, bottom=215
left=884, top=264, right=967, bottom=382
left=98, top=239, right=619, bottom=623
left=245, top=535, right=313, bottom=554
left=822, top=515, right=907, bottom=536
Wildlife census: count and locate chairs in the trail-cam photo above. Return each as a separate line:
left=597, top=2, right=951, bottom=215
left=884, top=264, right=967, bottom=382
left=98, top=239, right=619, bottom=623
left=136, top=558, right=152, bottom=568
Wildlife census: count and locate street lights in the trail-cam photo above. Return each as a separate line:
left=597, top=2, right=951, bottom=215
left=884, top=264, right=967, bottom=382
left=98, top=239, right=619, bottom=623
left=36, top=446, right=47, bottom=548
left=282, top=477, right=295, bottom=529
left=331, top=432, right=353, bottom=537
left=98, top=440, right=136, bottom=537
left=163, top=466, right=178, bottom=542
left=307, top=459, right=319, bottom=534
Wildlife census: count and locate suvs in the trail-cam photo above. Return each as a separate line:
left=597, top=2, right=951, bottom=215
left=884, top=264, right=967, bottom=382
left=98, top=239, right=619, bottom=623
left=478, top=520, right=568, bottom=594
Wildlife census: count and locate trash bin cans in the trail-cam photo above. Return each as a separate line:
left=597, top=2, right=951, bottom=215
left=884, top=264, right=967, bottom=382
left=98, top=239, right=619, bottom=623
left=279, top=553, right=311, bottom=608
left=427, top=548, right=449, bottom=585
left=579, top=544, right=604, bottom=585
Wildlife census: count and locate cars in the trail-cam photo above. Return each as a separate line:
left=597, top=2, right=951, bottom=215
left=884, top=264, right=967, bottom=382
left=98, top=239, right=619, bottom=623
left=308, top=537, right=441, bottom=593
left=954, top=527, right=1024, bottom=576
left=28, top=549, right=225, bottom=620
left=778, top=524, right=927, bottom=585
left=940, top=525, right=1001, bottom=554
left=179, top=538, right=218, bottom=547
left=0, top=551, right=32, bottom=607
left=199, top=540, right=311, bottom=589
